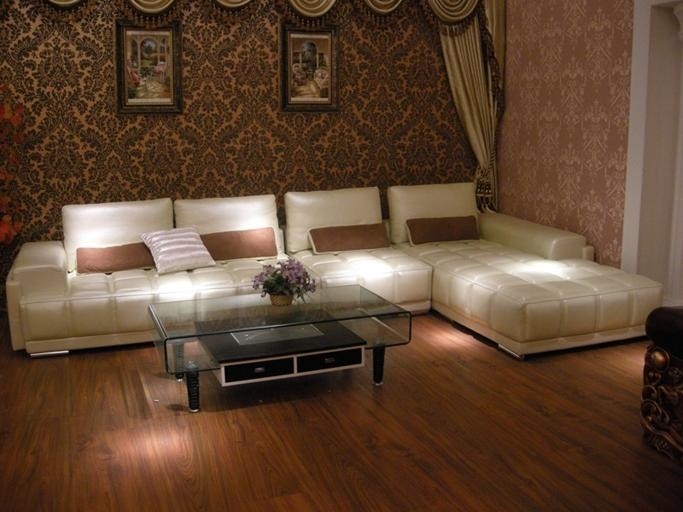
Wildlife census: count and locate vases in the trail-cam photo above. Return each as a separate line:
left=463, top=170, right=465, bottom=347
left=270, top=294, right=293, bottom=307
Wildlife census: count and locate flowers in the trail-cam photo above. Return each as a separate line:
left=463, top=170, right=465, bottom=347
left=251, top=257, right=316, bottom=303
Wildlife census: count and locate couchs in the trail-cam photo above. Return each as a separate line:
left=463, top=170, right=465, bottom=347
left=5, top=186, right=432, bottom=358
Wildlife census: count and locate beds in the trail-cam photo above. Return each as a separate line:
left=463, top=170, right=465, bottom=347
left=384, top=181, right=664, bottom=361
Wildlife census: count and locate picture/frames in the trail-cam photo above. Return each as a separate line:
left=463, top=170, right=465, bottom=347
left=115, top=19, right=183, bottom=115
left=280, top=19, right=339, bottom=112
